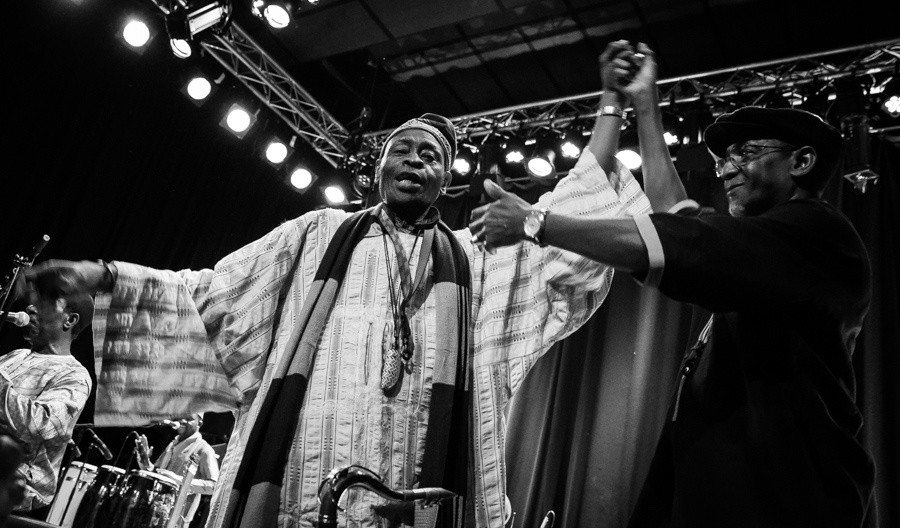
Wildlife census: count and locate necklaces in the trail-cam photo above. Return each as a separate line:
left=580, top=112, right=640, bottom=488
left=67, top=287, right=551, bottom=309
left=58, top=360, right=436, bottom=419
left=671, top=312, right=713, bottom=421
left=378, top=222, right=420, bottom=391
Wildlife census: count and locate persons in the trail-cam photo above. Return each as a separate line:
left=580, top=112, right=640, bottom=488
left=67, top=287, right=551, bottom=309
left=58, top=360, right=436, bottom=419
left=20, top=38, right=669, bottom=528
left=0, top=292, right=95, bottom=517
left=469, top=42, right=881, bottom=528
left=135, top=411, right=220, bottom=528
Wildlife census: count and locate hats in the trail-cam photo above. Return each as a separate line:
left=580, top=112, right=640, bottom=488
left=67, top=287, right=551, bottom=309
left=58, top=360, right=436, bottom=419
left=702, top=104, right=844, bottom=160
left=380, top=113, right=457, bottom=171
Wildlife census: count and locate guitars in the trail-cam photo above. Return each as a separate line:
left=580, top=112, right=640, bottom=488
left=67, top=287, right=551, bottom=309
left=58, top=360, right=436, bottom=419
left=164, top=453, right=201, bottom=528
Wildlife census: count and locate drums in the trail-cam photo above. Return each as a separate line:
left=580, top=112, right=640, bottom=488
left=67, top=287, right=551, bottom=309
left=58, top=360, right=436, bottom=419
left=72, top=464, right=126, bottom=528
left=117, top=469, right=179, bottom=528
left=46, top=460, right=98, bottom=528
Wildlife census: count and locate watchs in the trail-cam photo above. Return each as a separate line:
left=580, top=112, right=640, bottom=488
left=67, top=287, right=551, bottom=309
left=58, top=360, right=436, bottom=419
left=523, top=207, right=551, bottom=247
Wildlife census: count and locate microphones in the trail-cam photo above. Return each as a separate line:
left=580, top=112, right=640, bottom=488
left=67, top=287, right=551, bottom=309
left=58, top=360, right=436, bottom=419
left=0, top=311, right=30, bottom=327
left=89, top=429, right=113, bottom=460
left=164, top=420, right=180, bottom=430
left=70, top=439, right=82, bottom=458
left=133, top=432, right=154, bottom=471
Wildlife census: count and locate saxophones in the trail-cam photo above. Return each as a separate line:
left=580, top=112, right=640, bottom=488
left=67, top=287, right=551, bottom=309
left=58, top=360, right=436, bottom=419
left=311, top=465, right=457, bottom=528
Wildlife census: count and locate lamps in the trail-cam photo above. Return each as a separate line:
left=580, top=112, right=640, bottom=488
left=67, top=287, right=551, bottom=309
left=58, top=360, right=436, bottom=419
left=351, top=166, right=378, bottom=198
left=441, top=104, right=704, bottom=183
left=112, top=0, right=349, bottom=208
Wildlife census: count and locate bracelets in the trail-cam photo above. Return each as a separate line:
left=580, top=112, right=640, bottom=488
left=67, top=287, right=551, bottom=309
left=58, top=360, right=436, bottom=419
left=100, top=260, right=115, bottom=292
left=596, top=107, right=628, bottom=120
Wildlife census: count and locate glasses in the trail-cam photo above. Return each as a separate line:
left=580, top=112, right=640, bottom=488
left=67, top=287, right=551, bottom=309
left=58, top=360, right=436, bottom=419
left=714, top=142, right=795, bottom=178
